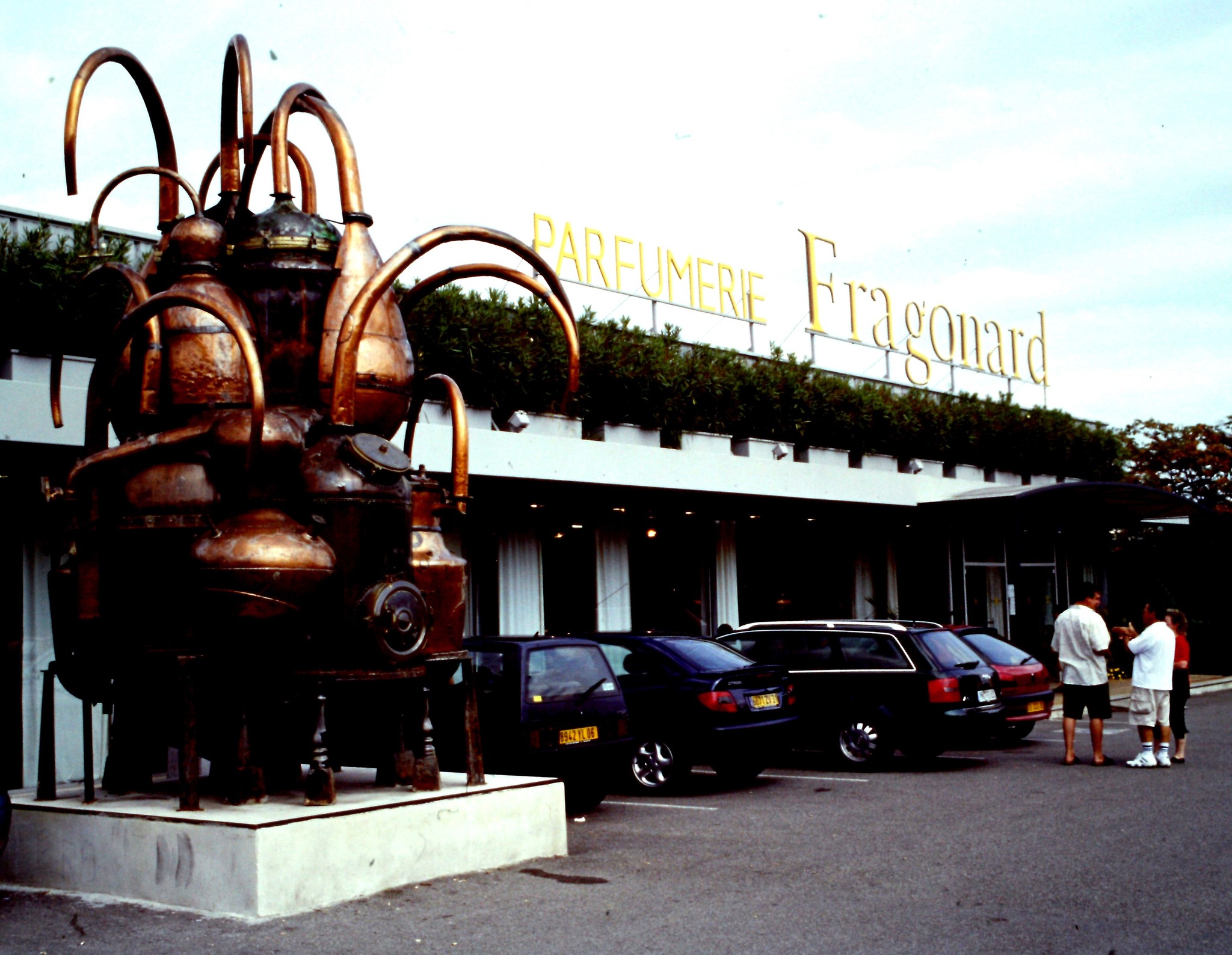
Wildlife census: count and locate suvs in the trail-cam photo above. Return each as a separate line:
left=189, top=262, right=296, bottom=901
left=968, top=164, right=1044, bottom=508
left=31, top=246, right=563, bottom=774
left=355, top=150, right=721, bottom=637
left=686, top=619, right=1055, bottom=772
left=430, top=631, right=628, bottom=818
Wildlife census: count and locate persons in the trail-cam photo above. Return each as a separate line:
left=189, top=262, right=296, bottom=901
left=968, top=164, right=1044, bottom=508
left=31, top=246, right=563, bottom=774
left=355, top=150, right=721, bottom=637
left=1051, top=584, right=1117, bottom=765
left=1153, top=607, right=1191, bottom=764
left=1113, top=601, right=1178, bottom=767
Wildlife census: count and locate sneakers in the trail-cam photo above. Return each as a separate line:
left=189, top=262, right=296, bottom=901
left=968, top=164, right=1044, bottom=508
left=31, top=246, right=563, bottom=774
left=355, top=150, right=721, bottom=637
left=1126, top=753, right=1158, bottom=768
left=1155, top=750, right=1172, bottom=767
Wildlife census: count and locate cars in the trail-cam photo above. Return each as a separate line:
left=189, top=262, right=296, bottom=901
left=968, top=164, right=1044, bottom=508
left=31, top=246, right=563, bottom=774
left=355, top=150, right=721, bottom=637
left=529, top=634, right=797, bottom=798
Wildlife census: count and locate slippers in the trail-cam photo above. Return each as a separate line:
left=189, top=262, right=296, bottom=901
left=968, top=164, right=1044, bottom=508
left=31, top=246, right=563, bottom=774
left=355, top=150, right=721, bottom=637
left=1095, top=754, right=1116, bottom=766
left=1063, top=755, right=1083, bottom=765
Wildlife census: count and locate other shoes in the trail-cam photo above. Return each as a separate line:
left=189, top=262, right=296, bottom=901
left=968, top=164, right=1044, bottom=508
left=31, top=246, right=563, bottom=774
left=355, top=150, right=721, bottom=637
left=1170, top=755, right=1186, bottom=764
